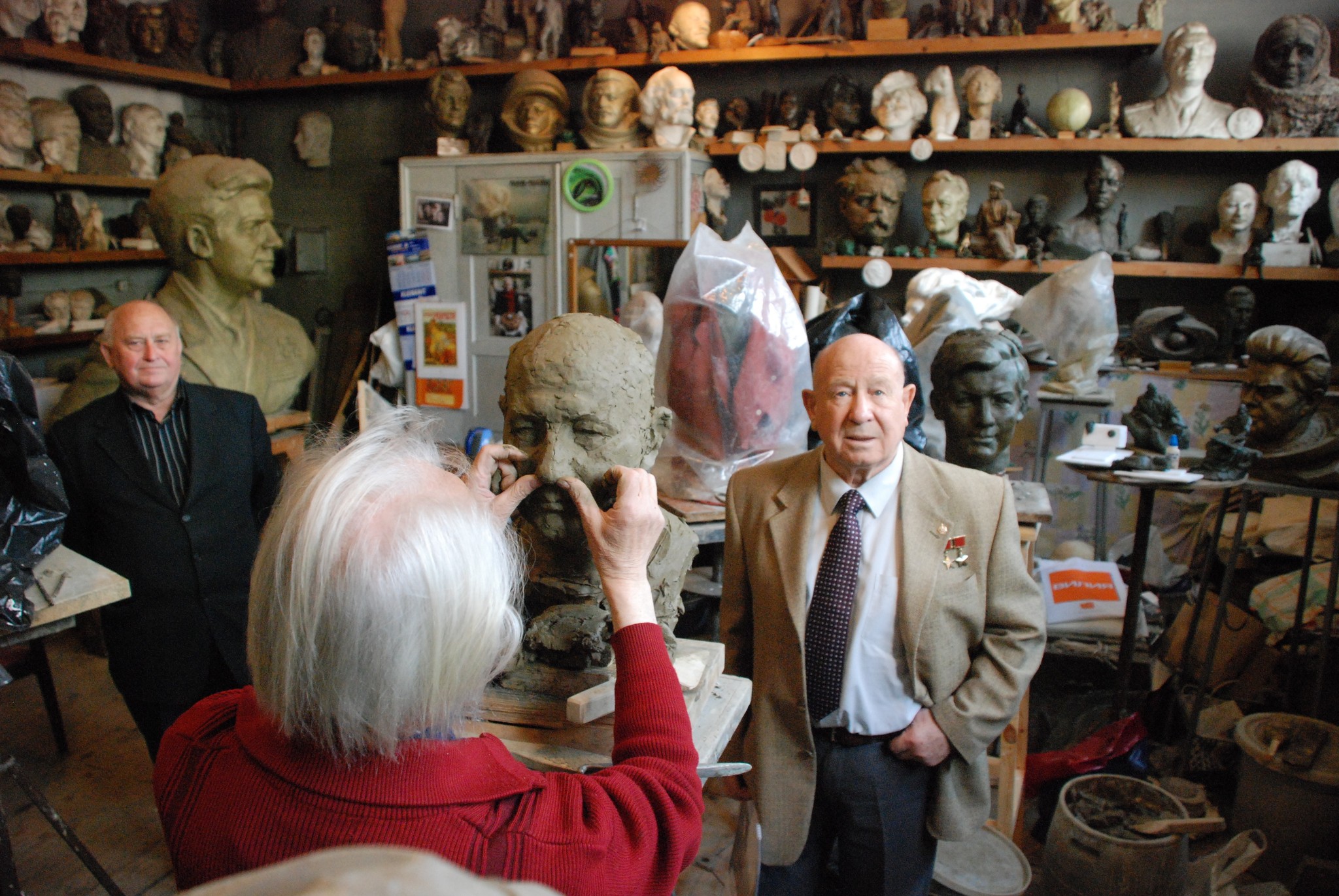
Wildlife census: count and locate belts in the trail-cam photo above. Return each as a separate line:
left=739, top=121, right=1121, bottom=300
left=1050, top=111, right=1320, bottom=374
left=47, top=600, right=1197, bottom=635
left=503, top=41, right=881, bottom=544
left=816, top=729, right=906, bottom=748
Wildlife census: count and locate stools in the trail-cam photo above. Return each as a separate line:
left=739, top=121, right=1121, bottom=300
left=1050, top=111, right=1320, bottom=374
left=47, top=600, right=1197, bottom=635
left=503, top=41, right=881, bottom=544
left=1035, top=385, right=1116, bottom=560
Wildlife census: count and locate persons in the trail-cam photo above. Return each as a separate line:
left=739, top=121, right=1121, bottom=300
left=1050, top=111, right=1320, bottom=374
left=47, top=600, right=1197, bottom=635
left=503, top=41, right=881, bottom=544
left=713, top=333, right=1047, bottom=896
left=48, top=154, right=318, bottom=425
left=152, top=407, right=707, bottom=896
left=495, top=312, right=700, bottom=665
left=46, top=299, right=284, bottom=767
left=0, top=78, right=203, bottom=338
left=491, top=276, right=521, bottom=315
left=483, top=205, right=533, bottom=253
left=1239, top=13, right=1339, bottom=136
left=406, top=0, right=1163, bottom=260
left=1211, top=160, right=1339, bottom=267
left=1131, top=287, right=1332, bottom=454
left=1, top=0, right=404, bottom=81
left=1123, top=22, right=1237, bottom=140
left=930, top=328, right=1032, bottom=477
left=294, top=111, right=332, bottom=168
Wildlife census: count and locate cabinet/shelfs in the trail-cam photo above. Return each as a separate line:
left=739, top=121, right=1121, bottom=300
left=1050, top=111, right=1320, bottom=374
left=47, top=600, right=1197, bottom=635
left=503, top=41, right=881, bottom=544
left=0, top=29, right=1339, bottom=358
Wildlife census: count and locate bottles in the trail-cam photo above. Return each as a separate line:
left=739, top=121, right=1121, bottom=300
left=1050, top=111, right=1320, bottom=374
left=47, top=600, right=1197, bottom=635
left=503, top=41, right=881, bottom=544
left=1165, top=434, right=1180, bottom=472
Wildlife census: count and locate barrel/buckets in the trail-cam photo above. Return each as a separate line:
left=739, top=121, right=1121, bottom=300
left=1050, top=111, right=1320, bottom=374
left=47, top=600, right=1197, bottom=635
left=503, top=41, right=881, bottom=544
left=1232, top=712, right=1339, bottom=896
left=927, top=822, right=1032, bottom=896
left=1040, top=773, right=1189, bottom=896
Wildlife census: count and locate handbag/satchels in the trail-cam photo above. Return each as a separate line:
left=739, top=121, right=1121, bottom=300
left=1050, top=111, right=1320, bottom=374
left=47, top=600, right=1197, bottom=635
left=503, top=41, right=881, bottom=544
left=1035, top=554, right=1127, bottom=625
left=1160, top=583, right=1262, bottom=690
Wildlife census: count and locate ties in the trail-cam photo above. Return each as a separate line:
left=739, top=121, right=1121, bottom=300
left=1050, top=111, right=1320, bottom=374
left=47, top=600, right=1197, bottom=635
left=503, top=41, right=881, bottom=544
left=805, top=490, right=866, bottom=723
